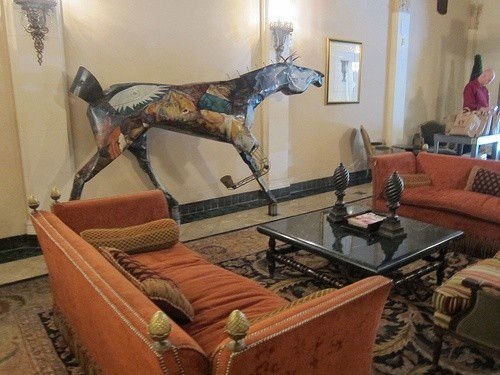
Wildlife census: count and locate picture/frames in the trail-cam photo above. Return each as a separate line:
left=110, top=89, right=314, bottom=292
left=324, top=37, right=363, bottom=106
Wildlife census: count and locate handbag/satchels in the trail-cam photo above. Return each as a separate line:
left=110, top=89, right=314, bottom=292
left=448, top=106, right=500, bottom=137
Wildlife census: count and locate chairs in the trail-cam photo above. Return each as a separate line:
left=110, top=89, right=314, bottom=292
left=360, top=125, right=396, bottom=174
left=430, top=252, right=500, bottom=371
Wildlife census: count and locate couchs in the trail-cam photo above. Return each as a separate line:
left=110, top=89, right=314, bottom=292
left=28, top=188, right=394, bottom=375
left=369, top=152, right=500, bottom=251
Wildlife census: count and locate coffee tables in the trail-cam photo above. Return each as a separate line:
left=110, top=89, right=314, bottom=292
left=257, top=205, right=464, bottom=322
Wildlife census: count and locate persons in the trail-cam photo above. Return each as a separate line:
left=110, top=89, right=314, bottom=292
left=463, top=68, right=495, bottom=112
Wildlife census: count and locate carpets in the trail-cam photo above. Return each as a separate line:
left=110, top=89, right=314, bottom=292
left=0, top=195, right=500, bottom=375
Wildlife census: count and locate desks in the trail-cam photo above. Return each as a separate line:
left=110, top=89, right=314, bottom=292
left=433, top=133, right=500, bottom=163
left=399, top=145, right=431, bottom=153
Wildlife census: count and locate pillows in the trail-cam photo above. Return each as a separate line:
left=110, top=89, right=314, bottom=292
left=99, top=245, right=193, bottom=323
left=464, top=165, right=500, bottom=197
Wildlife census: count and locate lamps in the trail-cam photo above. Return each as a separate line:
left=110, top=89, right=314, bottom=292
left=268, top=0, right=294, bottom=56
